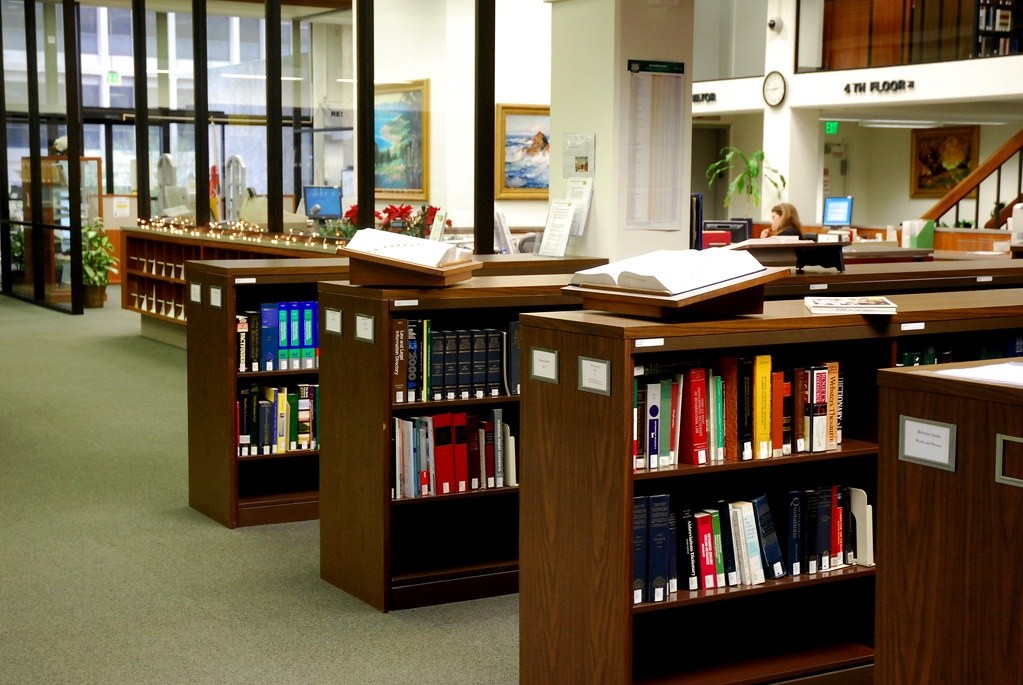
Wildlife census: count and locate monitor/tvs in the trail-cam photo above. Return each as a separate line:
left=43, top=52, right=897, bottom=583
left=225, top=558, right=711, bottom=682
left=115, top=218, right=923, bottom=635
left=702, top=219, right=749, bottom=244
left=302, top=185, right=342, bottom=226
left=822, top=196, right=853, bottom=231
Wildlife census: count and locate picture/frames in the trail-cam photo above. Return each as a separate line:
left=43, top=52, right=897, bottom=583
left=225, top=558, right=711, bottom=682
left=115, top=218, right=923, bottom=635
left=374, top=78, right=431, bottom=202
left=494, top=102, right=550, bottom=201
left=909, top=125, right=980, bottom=199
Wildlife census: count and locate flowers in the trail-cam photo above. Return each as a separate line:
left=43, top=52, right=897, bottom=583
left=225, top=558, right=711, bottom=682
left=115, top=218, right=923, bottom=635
left=344, top=203, right=453, bottom=239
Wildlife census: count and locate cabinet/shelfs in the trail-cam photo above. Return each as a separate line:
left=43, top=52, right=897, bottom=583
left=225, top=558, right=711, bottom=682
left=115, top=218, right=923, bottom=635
left=518, top=287, right=1023, bottom=685
left=318, top=260, right=1023, bottom=614
left=118, top=225, right=351, bottom=349
left=21, top=156, right=108, bottom=304
left=184, top=247, right=937, bottom=531
left=876, top=357, right=1023, bottom=685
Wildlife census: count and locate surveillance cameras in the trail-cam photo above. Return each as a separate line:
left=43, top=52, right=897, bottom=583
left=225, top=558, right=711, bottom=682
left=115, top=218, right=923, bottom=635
left=769, top=17, right=783, bottom=33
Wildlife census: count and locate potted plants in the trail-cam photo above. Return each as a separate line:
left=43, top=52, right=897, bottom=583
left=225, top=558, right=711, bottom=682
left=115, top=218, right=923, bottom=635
left=9, top=216, right=29, bottom=285
left=81, top=216, right=120, bottom=309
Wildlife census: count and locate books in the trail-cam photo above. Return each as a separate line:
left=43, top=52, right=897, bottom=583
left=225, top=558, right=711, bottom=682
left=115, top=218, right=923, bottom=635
left=897, top=333, right=1023, bottom=366
left=632, top=355, right=856, bottom=606
left=571, top=248, right=766, bottom=295
left=803, top=297, right=898, bottom=315
left=390, top=316, right=510, bottom=500
left=234, top=298, right=320, bottom=456
left=344, top=228, right=473, bottom=268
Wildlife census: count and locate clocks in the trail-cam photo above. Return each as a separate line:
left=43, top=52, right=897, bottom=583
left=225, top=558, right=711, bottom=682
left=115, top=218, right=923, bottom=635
left=762, top=71, right=786, bottom=107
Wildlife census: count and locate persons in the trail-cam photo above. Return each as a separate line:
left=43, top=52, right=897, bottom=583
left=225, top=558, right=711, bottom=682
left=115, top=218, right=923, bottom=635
left=761, top=203, right=803, bottom=240
